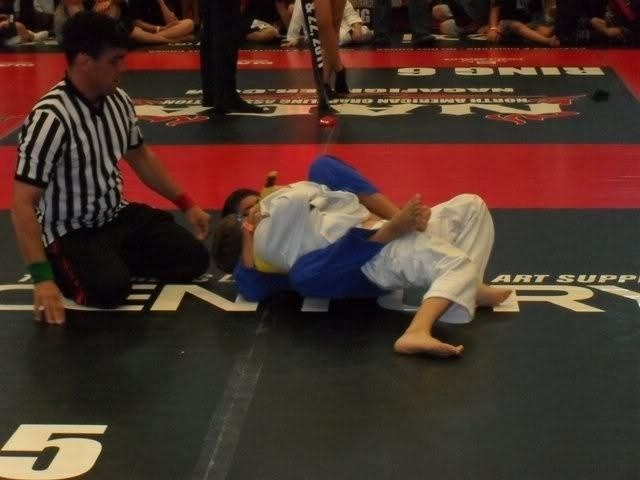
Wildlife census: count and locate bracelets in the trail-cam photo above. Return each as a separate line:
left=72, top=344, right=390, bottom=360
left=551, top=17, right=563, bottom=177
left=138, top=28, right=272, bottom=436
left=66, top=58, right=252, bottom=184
left=242, top=220, right=255, bottom=231
left=172, top=192, right=196, bottom=210
left=27, top=260, right=55, bottom=283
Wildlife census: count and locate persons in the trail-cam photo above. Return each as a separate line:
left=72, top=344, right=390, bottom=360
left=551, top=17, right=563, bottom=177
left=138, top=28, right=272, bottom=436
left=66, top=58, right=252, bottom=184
left=222, top=155, right=430, bottom=302
left=12, top=10, right=212, bottom=325
left=0, top=0, right=640, bottom=113
left=212, top=181, right=511, bottom=355
